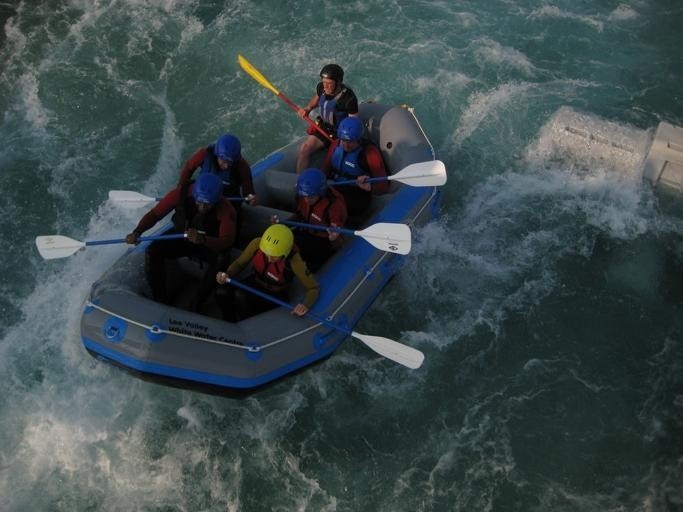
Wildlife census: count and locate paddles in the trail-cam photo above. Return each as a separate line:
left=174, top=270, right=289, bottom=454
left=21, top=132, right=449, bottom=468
left=238, top=54, right=335, bottom=144
left=274, top=216, right=412, bottom=256
left=222, top=273, right=425, bottom=370
left=36, top=229, right=206, bottom=259
left=326, top=160, right=447, bottom=188
left=108, top=190, right=249, bottom=210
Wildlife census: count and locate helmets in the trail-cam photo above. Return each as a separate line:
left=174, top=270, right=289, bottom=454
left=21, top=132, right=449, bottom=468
left=295, top=168, right=327, bottom=197
left=192, top=172, right=224, bottom=204
left=321, top=63, right=344, bottom=84
left=258, top=223, right=295, bottom=263
left=214, top=135, right=241, bottom=163
left=336, top=116, right=365, bottom=142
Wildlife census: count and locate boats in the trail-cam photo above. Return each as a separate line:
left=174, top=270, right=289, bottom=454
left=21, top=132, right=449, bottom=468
left=76, top=100, right=444, bottom=400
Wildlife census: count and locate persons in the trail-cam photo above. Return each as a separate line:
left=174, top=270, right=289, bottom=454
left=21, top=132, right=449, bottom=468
left=176, top=133, right=258, bottom=250
left=267, top=169, right=347, bottom=272
left=213, top=222, right=318, bottom=323
left=124, top=171, right=237, bottom=314
left=293, top=63, right=358, bottom=175
left=320, top=116, right=390, bottom=230
